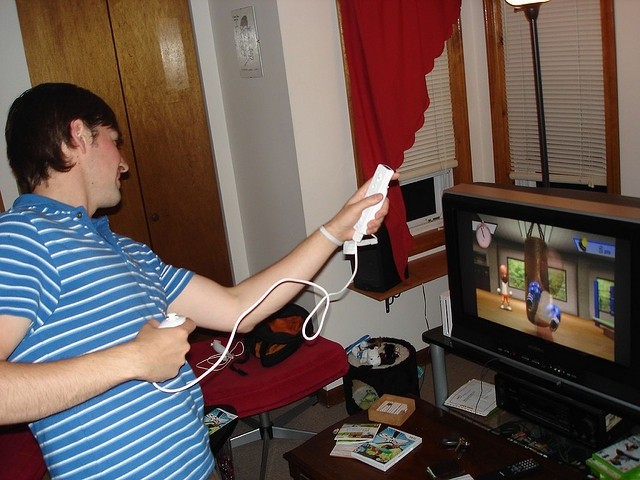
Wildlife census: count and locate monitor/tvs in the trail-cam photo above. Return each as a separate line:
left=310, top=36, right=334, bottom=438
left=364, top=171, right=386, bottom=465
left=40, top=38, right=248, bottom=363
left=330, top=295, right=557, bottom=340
left=445, top=186, right=637, bottom=400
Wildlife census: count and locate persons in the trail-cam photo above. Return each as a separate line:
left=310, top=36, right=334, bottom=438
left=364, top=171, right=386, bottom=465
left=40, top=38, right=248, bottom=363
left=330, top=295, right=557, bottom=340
left=0, top=83, right=400, bottom=479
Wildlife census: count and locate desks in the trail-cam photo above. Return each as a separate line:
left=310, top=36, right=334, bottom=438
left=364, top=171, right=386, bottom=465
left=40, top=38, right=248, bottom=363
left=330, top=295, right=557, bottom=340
left=182, top=333, right=349, bottom=480
left=418, top=326, right=639, bottom=459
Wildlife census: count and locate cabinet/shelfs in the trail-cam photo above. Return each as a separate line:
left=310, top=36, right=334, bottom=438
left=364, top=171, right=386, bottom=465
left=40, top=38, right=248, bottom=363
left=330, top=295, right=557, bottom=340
left=16, top=0, right=236, bottom=290
left=345, top=224, right=449, bottom=313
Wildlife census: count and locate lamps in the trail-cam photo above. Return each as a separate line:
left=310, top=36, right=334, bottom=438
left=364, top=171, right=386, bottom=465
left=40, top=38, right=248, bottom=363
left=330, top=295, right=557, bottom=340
left=504, top=0, right=558, bottom=22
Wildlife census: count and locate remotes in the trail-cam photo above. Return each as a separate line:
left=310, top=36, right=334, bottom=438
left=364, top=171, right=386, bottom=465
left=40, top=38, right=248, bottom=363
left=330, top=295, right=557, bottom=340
left=499, top=459, right=546, bottom=474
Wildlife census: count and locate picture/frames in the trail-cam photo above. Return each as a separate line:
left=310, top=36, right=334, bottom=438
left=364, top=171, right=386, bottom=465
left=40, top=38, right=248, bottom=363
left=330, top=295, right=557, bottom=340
left=231, top=5, right=265, bottom=79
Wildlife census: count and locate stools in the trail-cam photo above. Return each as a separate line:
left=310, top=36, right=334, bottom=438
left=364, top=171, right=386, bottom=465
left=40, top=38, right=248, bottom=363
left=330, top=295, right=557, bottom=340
left=343, top=338, right=417, bottom=416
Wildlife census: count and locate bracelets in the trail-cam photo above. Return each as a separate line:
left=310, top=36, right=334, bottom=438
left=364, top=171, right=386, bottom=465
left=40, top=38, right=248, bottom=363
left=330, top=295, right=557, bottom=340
left=317, top=224, right=344, bottom=246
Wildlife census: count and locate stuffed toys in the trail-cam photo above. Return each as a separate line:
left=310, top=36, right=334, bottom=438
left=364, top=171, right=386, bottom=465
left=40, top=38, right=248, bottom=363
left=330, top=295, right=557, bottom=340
left=351, top=341, right=381, bottom=366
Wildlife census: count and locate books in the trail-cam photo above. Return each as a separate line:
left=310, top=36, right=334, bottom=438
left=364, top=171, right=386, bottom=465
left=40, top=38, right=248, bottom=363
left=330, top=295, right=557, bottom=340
left=350, top=425, right=423, bottom=471
left=334, top=423, right=381, bottom=445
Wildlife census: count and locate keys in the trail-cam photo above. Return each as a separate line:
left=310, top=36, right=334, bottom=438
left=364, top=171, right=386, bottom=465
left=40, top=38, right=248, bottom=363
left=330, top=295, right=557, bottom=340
left=441, top=432, right=472, bottom=460
